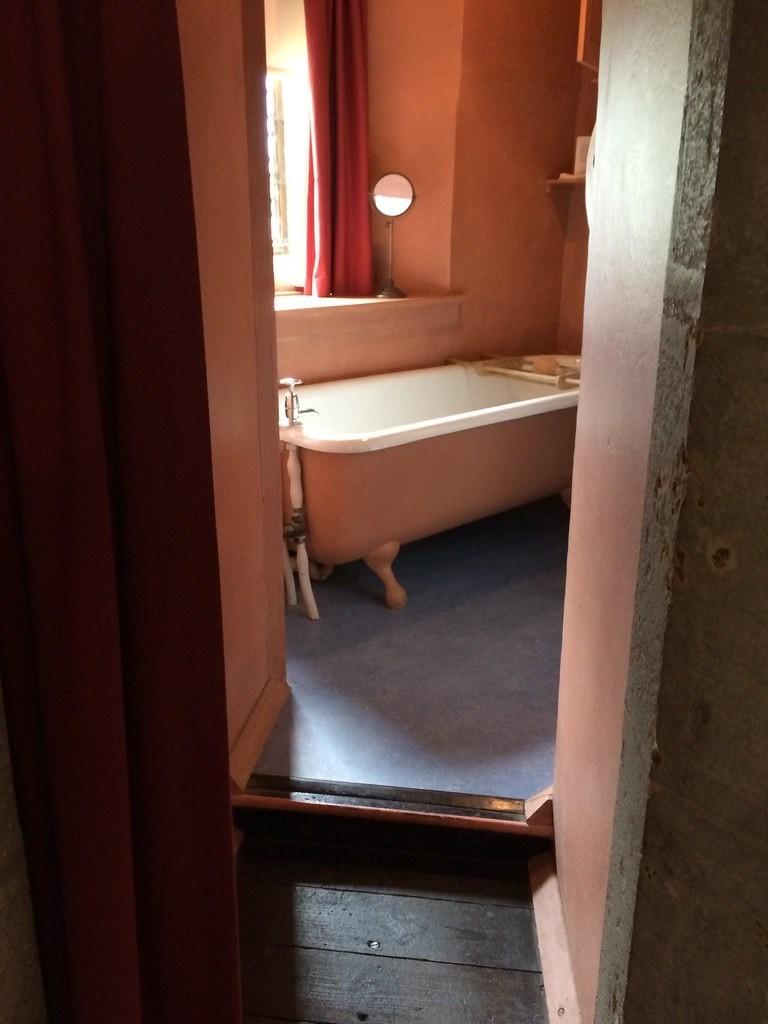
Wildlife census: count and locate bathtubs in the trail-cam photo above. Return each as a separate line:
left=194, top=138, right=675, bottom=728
left=278, top=352, right=582, bottom=607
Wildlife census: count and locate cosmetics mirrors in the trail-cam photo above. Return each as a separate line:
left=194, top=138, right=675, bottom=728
left=369, top=172, right=417, bottom=218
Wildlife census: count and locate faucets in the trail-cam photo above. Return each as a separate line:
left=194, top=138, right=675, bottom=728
left=280, top=378, right=321, bottom=422
